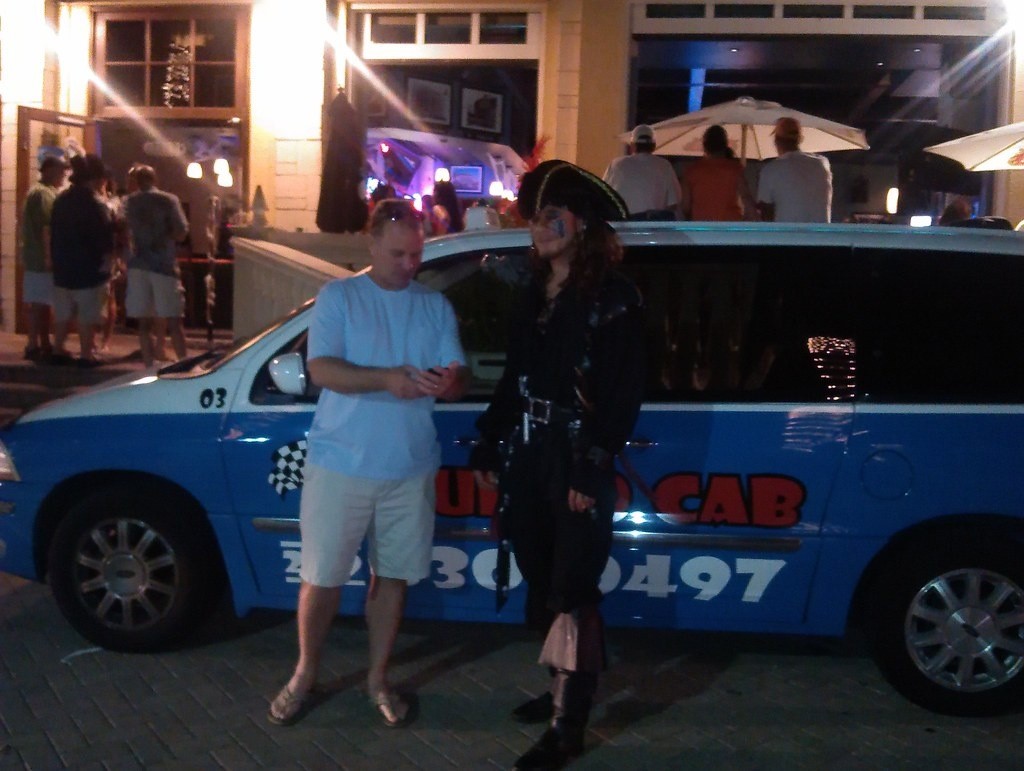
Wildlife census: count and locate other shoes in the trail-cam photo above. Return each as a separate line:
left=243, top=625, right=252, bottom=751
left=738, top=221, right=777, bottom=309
left=509, top=690, right=556, bottom=723
left=504, top=726, right=586, bottom=771
left=24, top=340, right=169, bottom=374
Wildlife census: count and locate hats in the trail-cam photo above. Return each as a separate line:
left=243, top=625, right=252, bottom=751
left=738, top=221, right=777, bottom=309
left=68, top=154, right=111, bottom=183
left=518, top=159, right=628, bottom=224
left=39, top=157, right=72, bottom=173
left=630, top=124, right=658, bottom=144
left=769, top=117, right=801, bottom=140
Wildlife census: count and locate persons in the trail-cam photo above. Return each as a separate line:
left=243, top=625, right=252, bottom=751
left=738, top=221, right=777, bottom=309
left=52, top=152, right=115, bottom=368
left=22, top=157, right=71, bottom=364
left=468, top=158, right=652, bottom=771
left=756, top=117, right=833, bottom=223
left=91, top=164, right=189, bottom=370
left=367, top=181, right=464, bottom=237
left=602, top=124, right=682, bottom=221
left=266, top=198, right=473, bottom=727
left=681, top=125, right=756, bottom=221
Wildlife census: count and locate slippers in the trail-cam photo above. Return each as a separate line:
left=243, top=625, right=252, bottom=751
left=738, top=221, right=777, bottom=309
left=266, top=680, right=323, bottom=725
left=362, top=672, right=411, bottom=728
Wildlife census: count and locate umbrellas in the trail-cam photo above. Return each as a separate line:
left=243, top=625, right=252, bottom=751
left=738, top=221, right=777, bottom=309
left=316, top=88, right=369, bottom=233
left=618, top=96, right=871, bottom=166
left=923, top=121, right=1024, bottom=171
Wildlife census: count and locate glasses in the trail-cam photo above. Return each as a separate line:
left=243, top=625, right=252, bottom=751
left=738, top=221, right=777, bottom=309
left=373, top=211, right=426, bottom=227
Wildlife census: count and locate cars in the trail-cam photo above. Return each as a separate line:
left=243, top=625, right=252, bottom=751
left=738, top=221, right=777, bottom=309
left=1, top=221, right=1023, bottom=720
